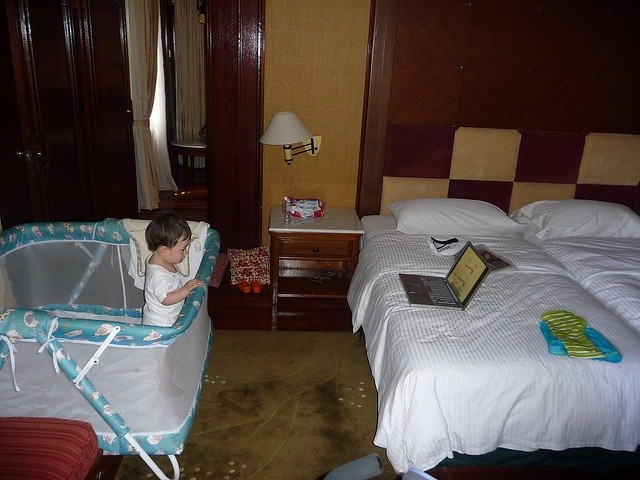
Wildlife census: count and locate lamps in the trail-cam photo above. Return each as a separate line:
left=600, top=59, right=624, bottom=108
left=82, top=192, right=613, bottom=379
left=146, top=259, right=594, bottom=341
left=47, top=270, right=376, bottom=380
left=260, top=110, right=315, bottom=164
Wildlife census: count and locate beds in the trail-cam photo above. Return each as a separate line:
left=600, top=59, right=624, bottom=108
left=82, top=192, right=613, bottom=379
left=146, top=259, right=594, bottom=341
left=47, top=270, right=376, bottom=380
left=346, top=213, right=640, bottom=480
left=1, top=219, right=222, bottom=479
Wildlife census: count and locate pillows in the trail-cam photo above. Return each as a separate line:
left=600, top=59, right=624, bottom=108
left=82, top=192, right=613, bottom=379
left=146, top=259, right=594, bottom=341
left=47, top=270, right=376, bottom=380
left=519, top=199, right=640, bottom=240
left=385, top=198, right=528, bottom=235
left=226, top=246, right=270, bottom=287
left=209, top=252, right=228, bottom=286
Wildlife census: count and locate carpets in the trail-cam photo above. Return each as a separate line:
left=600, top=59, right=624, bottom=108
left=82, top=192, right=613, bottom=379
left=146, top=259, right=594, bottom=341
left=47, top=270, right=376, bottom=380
left=113, top=327, right=398, bottom=479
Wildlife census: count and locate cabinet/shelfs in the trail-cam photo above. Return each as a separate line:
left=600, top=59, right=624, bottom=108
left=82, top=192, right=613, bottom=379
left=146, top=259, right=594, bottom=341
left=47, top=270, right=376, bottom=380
left=0, top=0, right=140, bottom=309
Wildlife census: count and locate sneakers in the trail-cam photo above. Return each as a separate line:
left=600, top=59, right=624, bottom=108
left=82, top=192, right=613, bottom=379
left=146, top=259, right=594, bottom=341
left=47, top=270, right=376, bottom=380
left=238, top=281, right=251, bottom=293
left=251, top=281, right=261, bottom=293
left=245, top=289, right=246, bottom=289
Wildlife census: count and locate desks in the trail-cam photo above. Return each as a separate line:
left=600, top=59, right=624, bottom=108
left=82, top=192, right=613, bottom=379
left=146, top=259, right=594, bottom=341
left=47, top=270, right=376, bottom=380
left=171, top=140, right=206, bottom=193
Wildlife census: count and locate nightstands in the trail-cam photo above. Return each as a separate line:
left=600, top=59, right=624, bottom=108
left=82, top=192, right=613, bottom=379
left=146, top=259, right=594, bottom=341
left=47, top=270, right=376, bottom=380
left=268, top=203, right=366, bottom=331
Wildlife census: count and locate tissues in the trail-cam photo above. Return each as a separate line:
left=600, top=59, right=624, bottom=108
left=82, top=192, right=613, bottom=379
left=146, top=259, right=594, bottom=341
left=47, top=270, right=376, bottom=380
left=284, top=195, right=325, bottom=218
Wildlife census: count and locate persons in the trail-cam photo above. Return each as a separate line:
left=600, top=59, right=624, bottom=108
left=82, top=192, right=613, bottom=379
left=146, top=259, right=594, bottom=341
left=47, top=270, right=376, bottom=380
left=140, top=211, right=205, bottom=398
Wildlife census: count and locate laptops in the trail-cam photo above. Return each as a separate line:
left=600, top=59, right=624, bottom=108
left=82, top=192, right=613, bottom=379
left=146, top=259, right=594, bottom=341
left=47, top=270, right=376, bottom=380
left=399, top=240, right=492, bottom=311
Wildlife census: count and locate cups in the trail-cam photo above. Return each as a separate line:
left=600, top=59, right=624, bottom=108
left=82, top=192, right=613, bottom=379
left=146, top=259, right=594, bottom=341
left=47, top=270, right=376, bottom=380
left=282, top=202, right=292, bottom=223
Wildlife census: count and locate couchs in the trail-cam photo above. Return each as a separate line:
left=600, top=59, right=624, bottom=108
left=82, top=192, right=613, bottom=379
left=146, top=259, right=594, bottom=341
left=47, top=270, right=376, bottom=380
left=0, top=417, right=104, bottom=479
